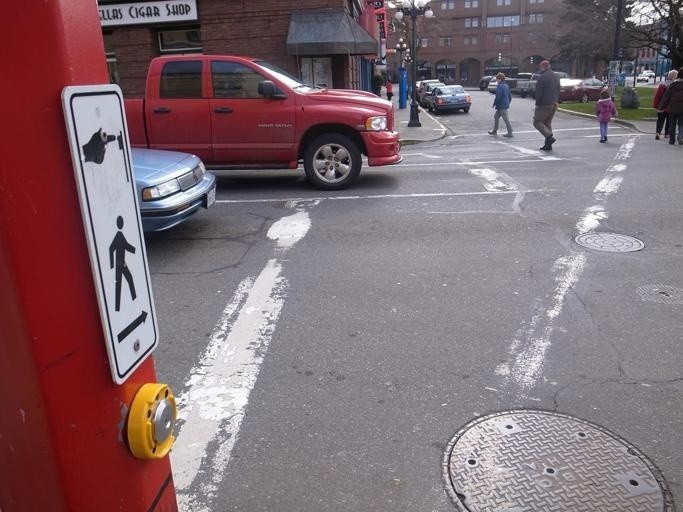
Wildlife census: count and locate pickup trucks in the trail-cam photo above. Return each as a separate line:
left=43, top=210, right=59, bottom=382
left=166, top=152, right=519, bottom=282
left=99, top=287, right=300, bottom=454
left=122, top=53, right=403, bottom=191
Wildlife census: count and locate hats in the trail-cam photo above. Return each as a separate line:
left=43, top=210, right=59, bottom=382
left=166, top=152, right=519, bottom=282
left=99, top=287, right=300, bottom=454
left=539, top=60, right=550, bottom=67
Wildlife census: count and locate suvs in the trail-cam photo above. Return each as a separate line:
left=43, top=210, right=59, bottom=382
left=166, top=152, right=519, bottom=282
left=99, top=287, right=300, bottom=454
left=642, top=69, right=655, bottom=79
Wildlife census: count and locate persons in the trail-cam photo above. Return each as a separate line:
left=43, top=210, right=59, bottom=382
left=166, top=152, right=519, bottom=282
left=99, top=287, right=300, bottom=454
left=658, top=68, right=683, bottom=145
left=385, top=79, right=394, bottom=100
left=488, top=72, right=513, bottom=137
left=596, top=90, right=619, bottom=142
left=532, top=60, right=560, bottom=150
left=652, top=70, right=678, bottom=139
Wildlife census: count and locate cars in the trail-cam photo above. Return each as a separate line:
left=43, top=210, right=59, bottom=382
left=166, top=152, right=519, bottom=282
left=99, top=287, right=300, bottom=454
left=479, top=71, right=608, bottom=103
left=636, top=73, right=649, bottom=83
left=415, top=71, right=471, bottom=116
left=128, top=148, right=216, bottom=232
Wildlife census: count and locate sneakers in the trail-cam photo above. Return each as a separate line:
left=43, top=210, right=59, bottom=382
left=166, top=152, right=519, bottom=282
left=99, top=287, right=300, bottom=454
left=665, top=134, right=670, bottom=138
left=545, top=136, right=556, bottom=149
left=600, top=136, right=607, bottom=142
left=503, top=134, right=513, bottom=138
left=488, top=131, right=497, bottom=134
left=655, top=133, right=660, bottom=140
left=541, top=146, right=553, bottom=150
left=677, top=136, right=683, bottom=145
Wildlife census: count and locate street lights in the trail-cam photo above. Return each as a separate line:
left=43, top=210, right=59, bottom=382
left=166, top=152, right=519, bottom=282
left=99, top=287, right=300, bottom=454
left=394, top=0, right=433, bottom=127
left=393, top=37, right=413, bottom=110
